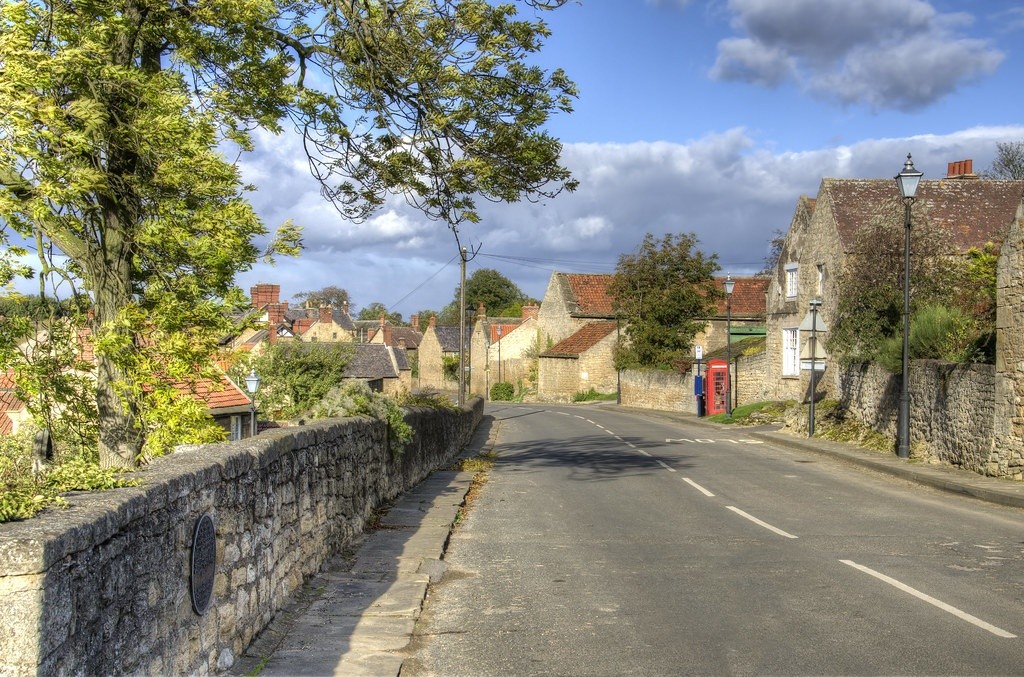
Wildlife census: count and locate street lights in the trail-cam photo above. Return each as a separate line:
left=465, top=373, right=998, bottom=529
left=466, top=305, right=477, bottom=395
left=892, top=152, right=923, bottom=458
left=495, top=323, right=502, bottom=387
left=244, top=368, right=261, bottom=439
left=723, top=271, right=736, bottom=417
